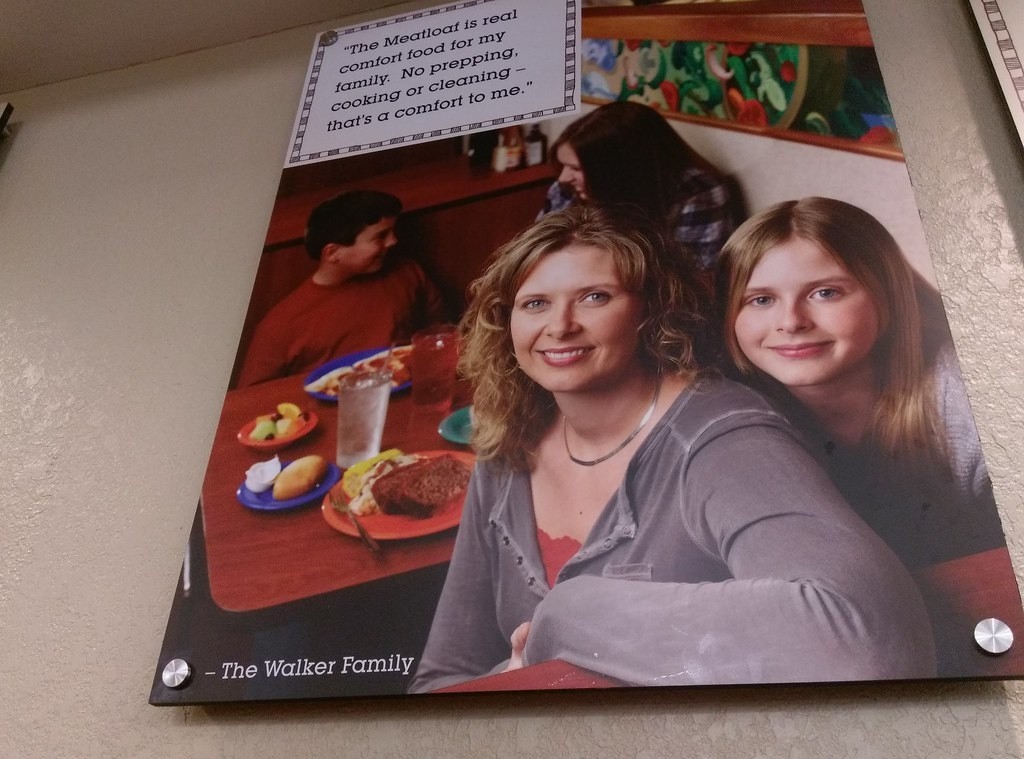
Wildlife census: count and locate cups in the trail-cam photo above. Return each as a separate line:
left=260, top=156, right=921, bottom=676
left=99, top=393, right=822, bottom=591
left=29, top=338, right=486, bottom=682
left=335, top=368, right=390, bottom=468
left=411, top=325, right=460, bottom=414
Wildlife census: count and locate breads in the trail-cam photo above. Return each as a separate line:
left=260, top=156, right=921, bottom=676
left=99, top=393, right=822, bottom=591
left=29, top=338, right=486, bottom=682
left=272, top=454, right=326, bottom=500
left=369, top=453, right=471, bottom=518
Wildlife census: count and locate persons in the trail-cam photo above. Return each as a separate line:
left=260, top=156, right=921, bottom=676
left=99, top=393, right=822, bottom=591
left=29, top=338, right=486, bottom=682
left=238, top=191, right=442, bottom=387
left=717, top=198, right=1005, bottom=548
left=534, top=102, right=732, bottom=271
left=409, top=204, right=937, bottom=695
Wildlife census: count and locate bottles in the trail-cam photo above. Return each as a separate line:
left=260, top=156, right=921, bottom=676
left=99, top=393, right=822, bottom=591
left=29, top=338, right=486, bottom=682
left=491, top=122, right=548, bottom=173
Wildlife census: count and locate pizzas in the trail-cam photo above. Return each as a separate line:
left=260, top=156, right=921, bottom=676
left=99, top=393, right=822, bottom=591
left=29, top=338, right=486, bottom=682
left=304, top=344, right=413, bottom=398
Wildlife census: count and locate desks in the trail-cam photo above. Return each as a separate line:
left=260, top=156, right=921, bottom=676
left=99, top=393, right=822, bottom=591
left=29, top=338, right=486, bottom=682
left=200, top=331, right=479, bottom=614
left=264, top=134, right=564, bottom=253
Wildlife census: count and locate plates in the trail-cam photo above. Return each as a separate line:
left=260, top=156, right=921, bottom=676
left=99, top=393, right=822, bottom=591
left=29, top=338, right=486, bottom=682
left=322, top=450, right=476, bottom=539
left=237, top=411, right=318, bottom=450
left=437, top=404, right=475, bottom=445
left=235, top=461, right=339, bottom=509
left=304, top=344, right=411, bottom=401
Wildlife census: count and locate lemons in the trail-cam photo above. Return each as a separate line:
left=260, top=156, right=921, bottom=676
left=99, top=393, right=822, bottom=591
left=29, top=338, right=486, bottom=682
left=276, top=402, right=302, bottom=418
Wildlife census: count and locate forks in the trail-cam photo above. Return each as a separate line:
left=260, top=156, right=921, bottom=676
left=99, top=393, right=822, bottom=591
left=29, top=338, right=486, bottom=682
left=328, top=484, right=384, bottom=561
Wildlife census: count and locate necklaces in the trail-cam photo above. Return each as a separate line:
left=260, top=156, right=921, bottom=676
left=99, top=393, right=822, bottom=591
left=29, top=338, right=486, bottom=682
left=562, top=369, right=662, bottom=466
